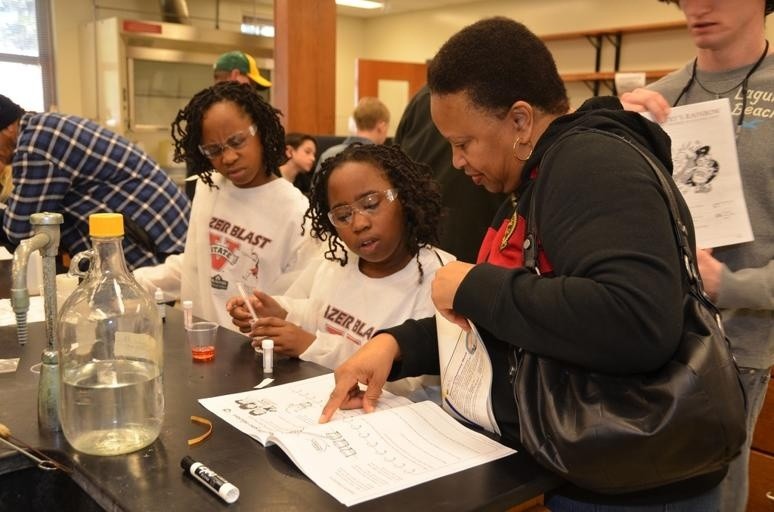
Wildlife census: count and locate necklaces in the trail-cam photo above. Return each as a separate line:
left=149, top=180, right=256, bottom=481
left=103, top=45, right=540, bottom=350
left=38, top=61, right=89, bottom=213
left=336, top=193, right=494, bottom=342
left=689, top=38, right=770, bottom=105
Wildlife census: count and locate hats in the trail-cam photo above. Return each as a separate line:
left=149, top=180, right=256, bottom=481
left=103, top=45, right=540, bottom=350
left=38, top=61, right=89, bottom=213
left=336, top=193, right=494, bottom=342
left=214, top=51, right=272, bottom=91
left=0, top=94, right=26, bottom=131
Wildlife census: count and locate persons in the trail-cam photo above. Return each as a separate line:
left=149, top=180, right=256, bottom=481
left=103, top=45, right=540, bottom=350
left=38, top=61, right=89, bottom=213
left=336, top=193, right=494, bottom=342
left=619, top=3, right=774, bottom=512
left=0, top=93, right=192, bottom=284
left=275, top=132, right=317, bottom=193
left=222, top=139, right=468, bottom=407
left=317, top=15, right=749, bottom=511
left=125, top=80, right=320, bottom=335
left=184, top=51, right=271, bottom=202
left=307, top=95, right=391, bottom=199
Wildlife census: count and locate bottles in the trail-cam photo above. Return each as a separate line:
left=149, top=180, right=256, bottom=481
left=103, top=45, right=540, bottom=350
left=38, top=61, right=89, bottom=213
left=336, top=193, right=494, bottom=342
left=154, top=288, right=166, bottom=324
left=56, top=212, right=164, bottom=458
left=183, top=300, right=193, bottom=331
left=261, top=339, right=274, bottom=374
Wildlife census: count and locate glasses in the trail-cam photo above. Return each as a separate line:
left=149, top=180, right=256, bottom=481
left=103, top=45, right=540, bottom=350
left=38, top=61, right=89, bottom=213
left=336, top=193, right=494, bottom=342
left=198, top=122, right=257, bottom=160
left=328, top=185, right=400, bottom=229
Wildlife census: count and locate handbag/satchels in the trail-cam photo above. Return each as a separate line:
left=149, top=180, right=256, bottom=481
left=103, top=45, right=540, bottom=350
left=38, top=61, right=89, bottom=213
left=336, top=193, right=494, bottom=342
left=512, top=289, right=748, bottom=494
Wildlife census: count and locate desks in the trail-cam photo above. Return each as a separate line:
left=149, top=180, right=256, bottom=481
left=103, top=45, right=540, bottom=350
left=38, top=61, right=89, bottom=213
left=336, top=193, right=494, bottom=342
left=0, top=258, right=560, bottom=511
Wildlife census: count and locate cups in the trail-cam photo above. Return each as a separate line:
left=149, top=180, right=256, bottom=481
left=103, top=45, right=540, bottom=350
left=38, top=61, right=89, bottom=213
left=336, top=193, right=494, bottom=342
left=249, top=316, right=266, bottom=354
left=185, top=320, right=220, bottom=360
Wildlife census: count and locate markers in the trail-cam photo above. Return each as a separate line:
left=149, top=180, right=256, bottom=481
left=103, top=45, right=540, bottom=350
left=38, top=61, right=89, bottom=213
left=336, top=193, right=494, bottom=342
left=181, top=455, right=240, bottom=504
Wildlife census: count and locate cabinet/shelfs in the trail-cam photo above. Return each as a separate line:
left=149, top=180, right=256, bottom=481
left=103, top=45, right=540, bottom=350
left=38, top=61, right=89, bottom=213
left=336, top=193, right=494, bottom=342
left=538, top=20, right=690, bottom=98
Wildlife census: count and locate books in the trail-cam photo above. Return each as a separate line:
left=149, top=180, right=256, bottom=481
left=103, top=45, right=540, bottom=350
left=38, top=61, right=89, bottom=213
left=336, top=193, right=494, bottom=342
left=198, top=370, right=521, bottom=511
left=431, top=299, right=501, bottom=440
